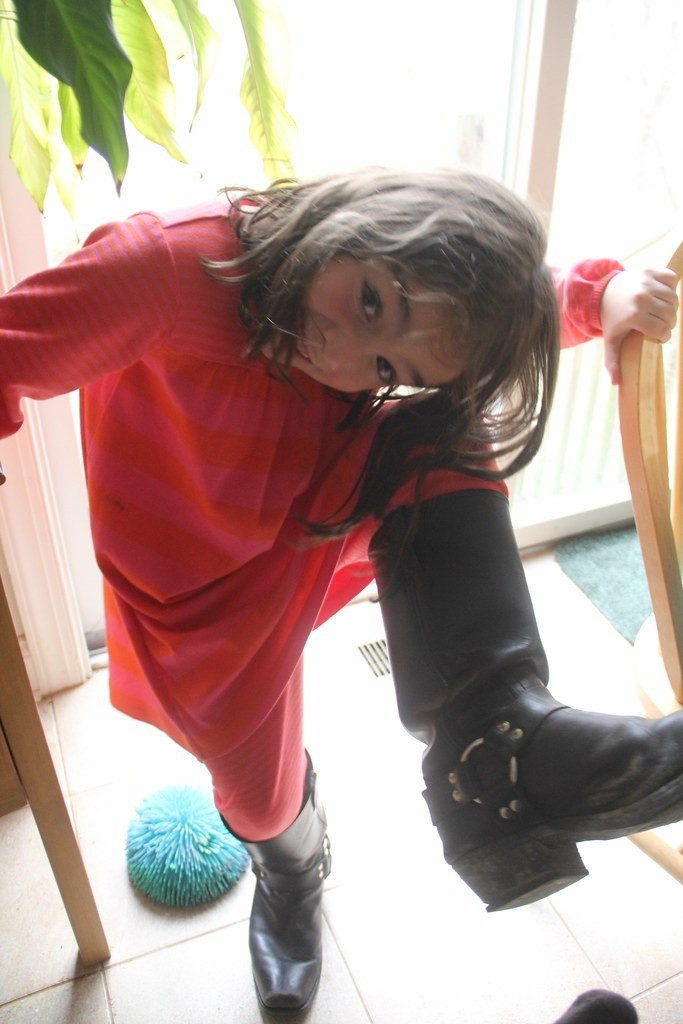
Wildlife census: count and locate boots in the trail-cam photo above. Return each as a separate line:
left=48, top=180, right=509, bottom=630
left=369, top=492, right=683, bottom=916
left=208, top=747, right=332, bottom=1021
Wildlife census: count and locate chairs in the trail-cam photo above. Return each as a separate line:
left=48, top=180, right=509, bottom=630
left=617, top=242, right=683, bottom=894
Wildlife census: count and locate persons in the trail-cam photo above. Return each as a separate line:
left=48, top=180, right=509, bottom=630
left=1, top=168, right=682, bottom=1024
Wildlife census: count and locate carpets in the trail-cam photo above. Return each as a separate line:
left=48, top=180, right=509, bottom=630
left=557, top=524, right=654, bottom=642
